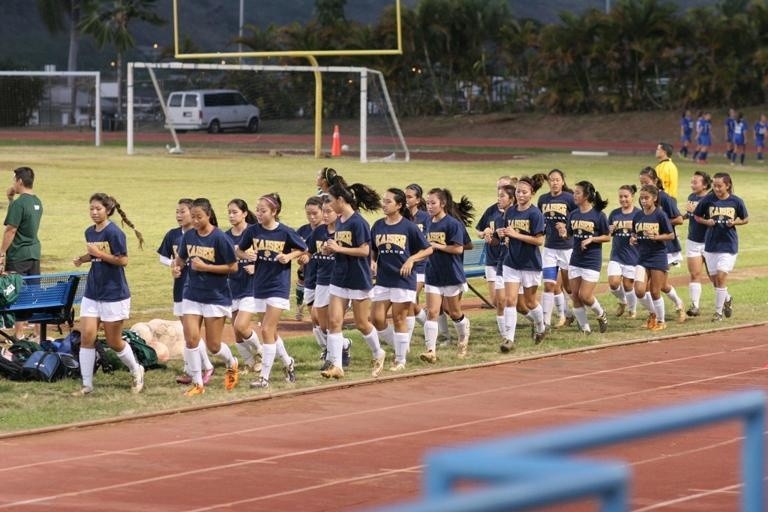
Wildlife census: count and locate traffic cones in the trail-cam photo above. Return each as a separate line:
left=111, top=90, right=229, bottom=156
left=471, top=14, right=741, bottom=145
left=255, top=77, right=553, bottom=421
left=330, top=124, right=341, bottom=158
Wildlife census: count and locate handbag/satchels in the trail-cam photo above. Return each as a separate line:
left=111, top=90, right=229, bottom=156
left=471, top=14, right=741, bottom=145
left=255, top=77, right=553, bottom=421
left=2, top=324, right=158, bottom=383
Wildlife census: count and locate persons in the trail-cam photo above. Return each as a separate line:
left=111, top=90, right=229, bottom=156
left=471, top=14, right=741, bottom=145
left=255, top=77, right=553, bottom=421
left=0, top=167, right=43, bottom=345
left=474, top=170, right=611, bottom=352
left=156, top=191, right=309, bottom=395
left=653, top=142, right=679, bottom=199
left=297, top=167, right=473, bottom=378
left=70, top=193, right=145, bottom=397
left=607, top=166, right=749, bottom=330
left=678, top=106, right=768, bottom=165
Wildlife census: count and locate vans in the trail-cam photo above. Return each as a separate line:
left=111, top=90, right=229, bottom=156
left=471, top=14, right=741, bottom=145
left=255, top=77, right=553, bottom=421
left=164, top=88, right=262, bottom=134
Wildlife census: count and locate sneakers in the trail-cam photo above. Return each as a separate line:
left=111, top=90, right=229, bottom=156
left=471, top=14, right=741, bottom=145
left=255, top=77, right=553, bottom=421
left=419, top=350, right=437, bottom=365
left=531, top=314, right=593, bottom=348
left=436, top=333, right=452, bottom=349
left=240, top=347, right=264, bottom=372
left=389, top=360, right=406, bottom=372
left=129, top=365, right=145, bottom=393
left=455, top=334, right=469, bottom=359
left=250, top=377, right=269, bottom=390
left=281, top=356, right=296, bottom=385
left=597, top=311, right=608, bottom=333
left=674, top=304, right=700, bottom=322
left=711, top=295, right=734, bottom=322
left=70, top=385, right=96, bottom=397
left=677, top=151, right=768, bottom=169
left=224, top=356, right=240, bottom=392
left=500, top=337, right=515, bottom=353
left=370, top=349, right=387, bottom=378
left=616, top=302, right=667, bottom=332
left=176, top=364, right=214, bottom=399
left=319, top=336, right=353, bottom=379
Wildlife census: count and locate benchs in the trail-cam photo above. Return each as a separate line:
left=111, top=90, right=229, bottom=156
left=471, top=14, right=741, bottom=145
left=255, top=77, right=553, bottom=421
left=463, top=242, right=496, bottom=310
left=0, top=271, right=89, bottom=344
